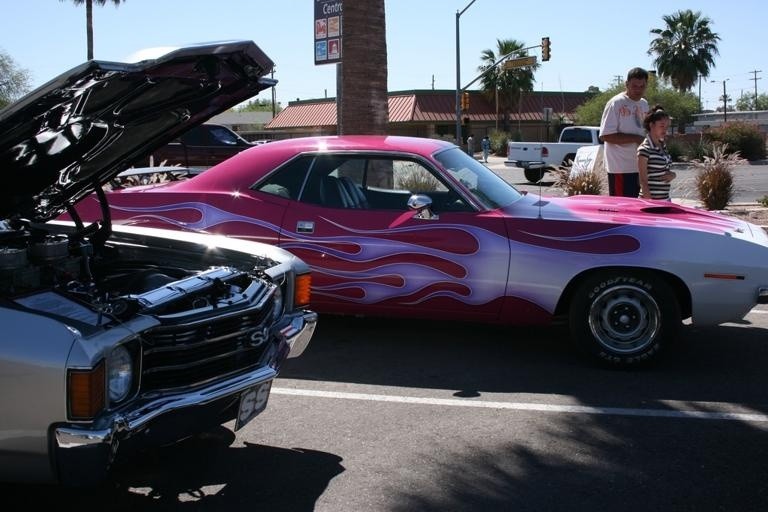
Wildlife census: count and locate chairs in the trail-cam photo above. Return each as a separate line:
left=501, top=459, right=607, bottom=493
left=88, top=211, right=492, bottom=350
left=319, top=176, right=355, bottom=209
left=338, top=177, right=371, bottom=209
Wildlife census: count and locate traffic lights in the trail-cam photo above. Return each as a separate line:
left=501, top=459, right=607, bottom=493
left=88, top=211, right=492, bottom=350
left=541, top=37, right=552, bottom=63
left=462, top=92, right=470, bottom=111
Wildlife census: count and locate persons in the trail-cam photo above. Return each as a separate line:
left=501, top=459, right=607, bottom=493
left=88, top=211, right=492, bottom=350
left=599, top=67, right=650, bottom=198
left=637, top=104, right=676, bottom=202
left=481, top=136, right=490, bottom=163
left=467, top=134, right=475, bottom=157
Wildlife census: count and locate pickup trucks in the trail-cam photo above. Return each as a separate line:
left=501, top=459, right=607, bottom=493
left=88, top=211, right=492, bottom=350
left=505, top=123, right=605, bottom=182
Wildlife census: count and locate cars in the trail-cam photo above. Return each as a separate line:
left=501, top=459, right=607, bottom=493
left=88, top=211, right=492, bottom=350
left=0, top=35, right=319, bottom=491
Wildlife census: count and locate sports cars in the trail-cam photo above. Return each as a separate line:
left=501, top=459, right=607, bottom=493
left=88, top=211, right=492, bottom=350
left=57, top=135, right=766, bottom=370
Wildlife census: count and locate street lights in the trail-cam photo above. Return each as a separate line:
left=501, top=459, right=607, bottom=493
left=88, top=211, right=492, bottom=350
left=709, top=77, right=731, bottom=122
